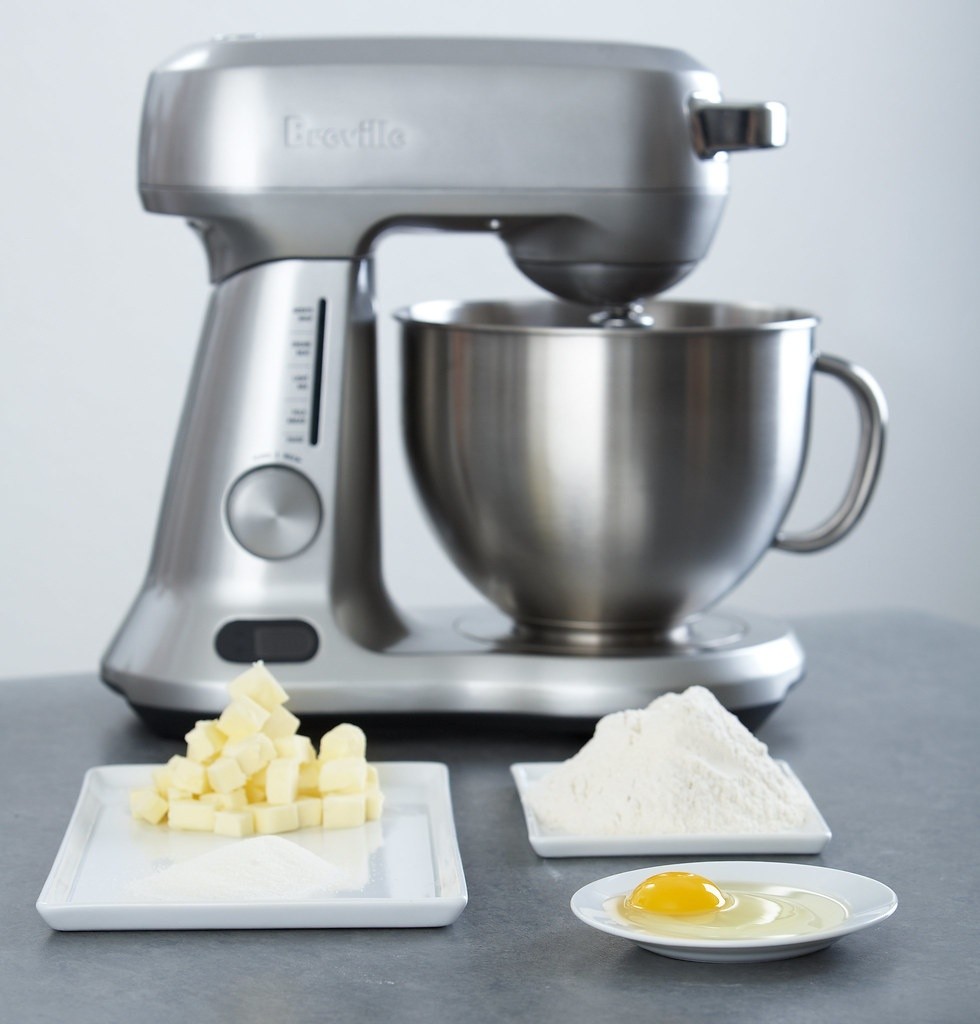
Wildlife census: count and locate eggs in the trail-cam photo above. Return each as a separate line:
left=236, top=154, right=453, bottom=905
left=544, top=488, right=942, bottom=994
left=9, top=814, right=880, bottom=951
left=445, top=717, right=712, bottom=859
left=604, top=870, right=850, bottom=938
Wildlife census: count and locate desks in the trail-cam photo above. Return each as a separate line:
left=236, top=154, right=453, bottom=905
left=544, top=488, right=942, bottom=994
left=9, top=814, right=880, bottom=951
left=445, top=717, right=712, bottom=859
left=0, top=604, right=980, bottom=1024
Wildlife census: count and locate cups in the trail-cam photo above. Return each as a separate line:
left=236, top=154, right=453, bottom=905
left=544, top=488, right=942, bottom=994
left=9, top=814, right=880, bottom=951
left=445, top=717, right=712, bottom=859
left=390, top=290, right=890, bottom=659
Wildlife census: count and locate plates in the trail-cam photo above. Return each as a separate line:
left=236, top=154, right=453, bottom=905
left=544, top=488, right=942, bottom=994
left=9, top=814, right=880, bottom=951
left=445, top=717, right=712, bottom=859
left=33, top=762, right=471, bottom=933
left=507, top=758, right=835, bottom=858
left=569, top=861, right=900, bottom=964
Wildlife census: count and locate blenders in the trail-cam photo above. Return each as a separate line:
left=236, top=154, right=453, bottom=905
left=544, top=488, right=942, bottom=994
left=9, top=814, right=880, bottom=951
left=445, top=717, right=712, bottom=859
left=94, top=31, right=810, bottom=753
